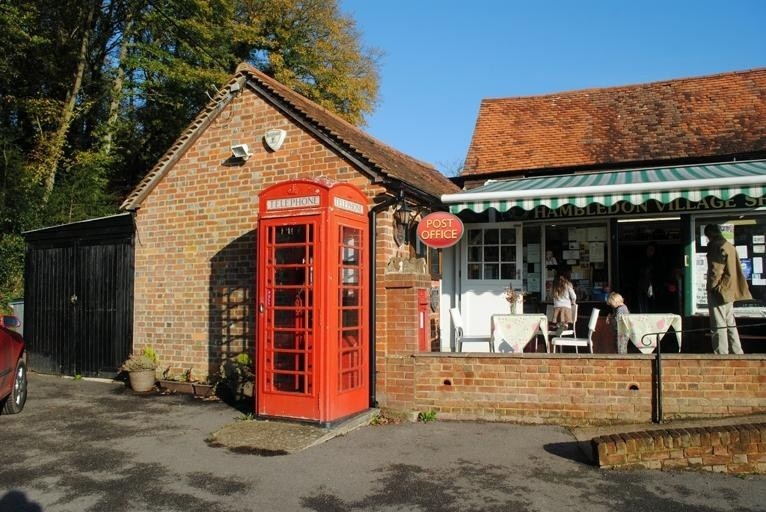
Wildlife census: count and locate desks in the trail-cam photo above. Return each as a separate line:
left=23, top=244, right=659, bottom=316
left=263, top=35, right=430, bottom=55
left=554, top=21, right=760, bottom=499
left=614, top=311, right=684, bottom=356
left=489, top=313, right=551, bottom=353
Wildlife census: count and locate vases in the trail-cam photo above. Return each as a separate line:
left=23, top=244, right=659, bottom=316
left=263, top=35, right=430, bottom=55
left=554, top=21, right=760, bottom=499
left=511, top=302, right=517, bottom=313
left=194, top=383, right=214, bottom=397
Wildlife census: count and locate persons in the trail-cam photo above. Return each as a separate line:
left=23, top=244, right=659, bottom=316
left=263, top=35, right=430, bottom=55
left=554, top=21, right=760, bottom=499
left=550, top=265, right=576, bottom=330
left=606, top=292, right=631, bottom=354
left=635, top=242, right=670, bottom=313
left=705, top=224, right=753, bottom=354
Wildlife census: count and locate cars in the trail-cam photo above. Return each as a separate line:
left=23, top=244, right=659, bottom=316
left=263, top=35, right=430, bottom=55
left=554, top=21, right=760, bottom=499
left=0, top=313, right=28, bottom=415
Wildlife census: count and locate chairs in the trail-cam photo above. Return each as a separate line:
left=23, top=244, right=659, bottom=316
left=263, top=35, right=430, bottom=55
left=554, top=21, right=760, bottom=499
left=550, top=304, right=580, bottom=353
left=535, top=304, right=556, bottom=352
left=551, top=307, right=601, bottom=354
left=449, top=307, right=494, bottom=353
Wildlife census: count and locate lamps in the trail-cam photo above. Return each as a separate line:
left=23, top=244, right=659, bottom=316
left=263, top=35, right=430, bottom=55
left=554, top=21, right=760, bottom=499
left=398, top=197, right=413, bottom=224
left=231, top=143, right=253, bottom=161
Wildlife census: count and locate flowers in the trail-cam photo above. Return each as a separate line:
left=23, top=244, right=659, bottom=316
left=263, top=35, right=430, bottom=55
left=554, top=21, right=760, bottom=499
left=504, top=282, right=532, bottom=302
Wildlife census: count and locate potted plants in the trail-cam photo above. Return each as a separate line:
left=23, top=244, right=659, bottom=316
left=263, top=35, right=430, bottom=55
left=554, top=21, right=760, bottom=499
left=160, top=365, right=199, bottom=394
left=115, top=347, right=158, bottom=394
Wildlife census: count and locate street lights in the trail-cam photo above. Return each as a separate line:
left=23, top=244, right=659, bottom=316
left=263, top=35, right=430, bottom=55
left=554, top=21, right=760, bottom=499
left=369, top=187, right=413, bottom=410
